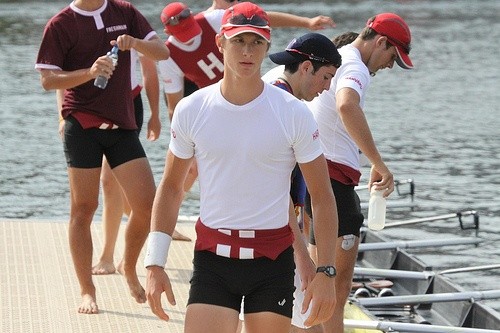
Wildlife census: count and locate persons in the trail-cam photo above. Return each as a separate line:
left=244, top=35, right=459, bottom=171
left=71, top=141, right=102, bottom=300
left=261, top=13, right=413, bottom=333
left=144, top=0, right=339, bottom=333
left=56, top=48, right=161, bottom=274
left=35, top=0, right=169, bottom=314
left=158, top=0, right=335, bottom=241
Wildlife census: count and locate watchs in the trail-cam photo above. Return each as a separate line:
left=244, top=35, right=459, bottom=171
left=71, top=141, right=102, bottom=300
left=317, top=266, right=337, bottom=278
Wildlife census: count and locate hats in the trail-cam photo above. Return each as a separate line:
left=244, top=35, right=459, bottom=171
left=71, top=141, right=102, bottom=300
left=269, top=32, right=342, bottom=68
left=219, top=2, right=271, bottom=42
left=161, top=2, right=202, bottom=44
left=365, top=12, right=415, bottom=70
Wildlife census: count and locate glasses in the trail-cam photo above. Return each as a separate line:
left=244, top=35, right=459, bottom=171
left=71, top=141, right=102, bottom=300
left=164, top=8, right=190, bottom=27
left=285, top=36, right=335, bottom=64
left=220, top=13, right=270, bottom=32
left=383, top=32, right=412, bottom=55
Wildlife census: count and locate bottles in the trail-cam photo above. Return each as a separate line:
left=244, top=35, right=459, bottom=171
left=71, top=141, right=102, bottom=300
left=94, top=40, right=118, bottom=89
left=367, top=178, right=386, bottom=231
